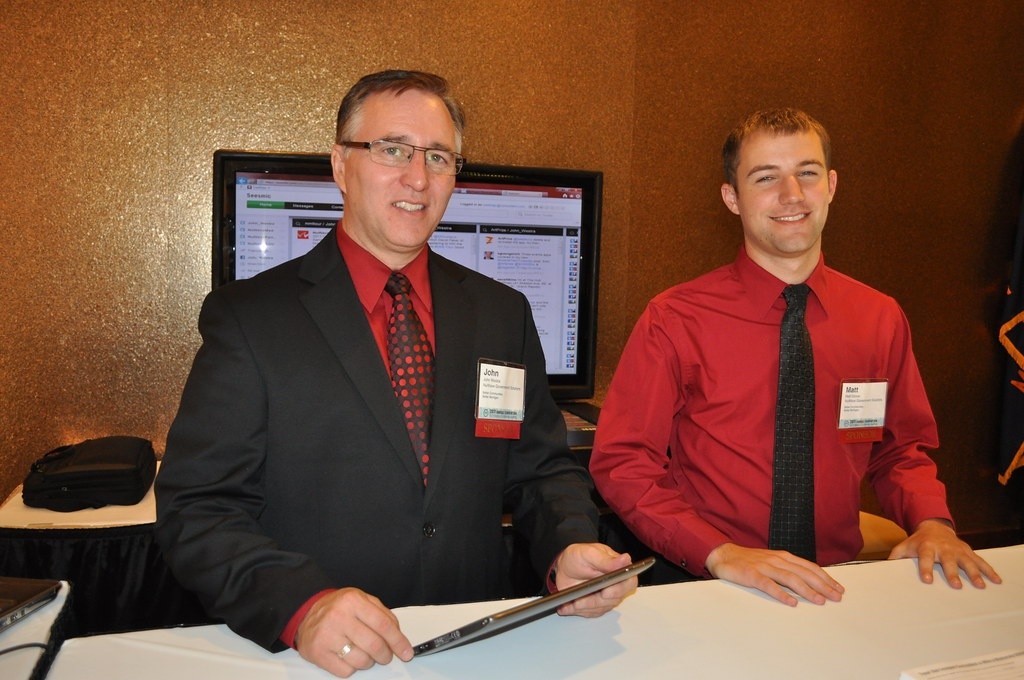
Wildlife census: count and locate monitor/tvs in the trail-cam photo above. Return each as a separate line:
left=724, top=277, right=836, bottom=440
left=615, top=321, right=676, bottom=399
left=215, top=148, right=604, bottom=400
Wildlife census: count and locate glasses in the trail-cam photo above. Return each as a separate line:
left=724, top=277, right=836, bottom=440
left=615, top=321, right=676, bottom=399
left=338, top=139, right=467, bottom=176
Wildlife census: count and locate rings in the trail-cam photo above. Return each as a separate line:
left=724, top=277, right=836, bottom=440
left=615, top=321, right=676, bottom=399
left=338, top=643, right=354, bottom=658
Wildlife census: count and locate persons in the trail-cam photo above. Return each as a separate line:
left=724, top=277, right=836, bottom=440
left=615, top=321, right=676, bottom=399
left=588, top=104, right=1002, bottom=606
left=155, top=69, right=639, bottom=679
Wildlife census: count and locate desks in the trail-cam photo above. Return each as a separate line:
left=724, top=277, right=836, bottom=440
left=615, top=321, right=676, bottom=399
left=0, top=458, right=161, bottom=529
left=0, top=573, right=69, bottom=680
left=48, top=541, right=1024, bottom=680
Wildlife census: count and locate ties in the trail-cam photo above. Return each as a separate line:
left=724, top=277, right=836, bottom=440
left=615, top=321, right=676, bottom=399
left=384, top=272, right=431, bottom=486
left=769, top=283, right=816, bottom=562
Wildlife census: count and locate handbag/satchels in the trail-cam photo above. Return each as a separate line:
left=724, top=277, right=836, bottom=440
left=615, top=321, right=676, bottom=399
left=22, top=436, right=157, bottom=512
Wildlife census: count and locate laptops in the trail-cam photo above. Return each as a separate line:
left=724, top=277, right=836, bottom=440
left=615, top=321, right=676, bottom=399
left=0, top=575, right=62, bottom=634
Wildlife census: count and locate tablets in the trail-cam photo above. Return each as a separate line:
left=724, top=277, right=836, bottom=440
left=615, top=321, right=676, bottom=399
left=412, top=555, right=656, bottom=656
